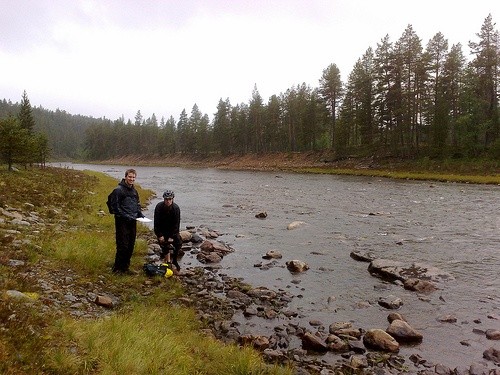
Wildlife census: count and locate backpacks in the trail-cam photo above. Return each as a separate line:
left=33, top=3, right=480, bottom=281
left=143, top=262, right=174, bottom=278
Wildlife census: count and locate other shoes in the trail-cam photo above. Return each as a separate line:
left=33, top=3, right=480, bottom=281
left=123, top=269, right=137, bottom=276
left=114, top=270, right=122, bottom=277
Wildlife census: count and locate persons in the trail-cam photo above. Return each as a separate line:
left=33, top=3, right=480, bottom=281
left=153, top=189, right=181, bottom=271
left=110, top=169, right=140, bottom=274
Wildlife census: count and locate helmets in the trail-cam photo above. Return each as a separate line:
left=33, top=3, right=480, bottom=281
left=163, top=190, right=175, bottom=198
